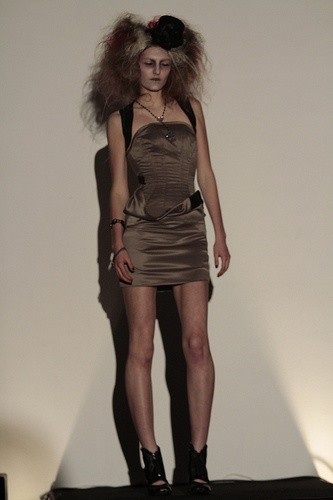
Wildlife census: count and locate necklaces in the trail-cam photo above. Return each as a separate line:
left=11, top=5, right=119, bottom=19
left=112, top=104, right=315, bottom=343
left=135, top=96, right=169, bottom=123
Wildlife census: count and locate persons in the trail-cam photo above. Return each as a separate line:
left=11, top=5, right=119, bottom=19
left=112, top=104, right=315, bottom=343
left=80, top=13, right=231, bottom=500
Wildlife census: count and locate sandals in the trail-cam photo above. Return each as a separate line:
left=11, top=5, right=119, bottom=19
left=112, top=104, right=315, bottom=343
left=189, top=444, right=212, bottom=495
left=141, top=447, right=172, bottom=494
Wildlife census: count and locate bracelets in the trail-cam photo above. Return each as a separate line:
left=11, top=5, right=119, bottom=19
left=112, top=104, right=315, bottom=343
left=112, top=248, right=128, bottom=262
left=108, top=217, right=126, bottom=230
